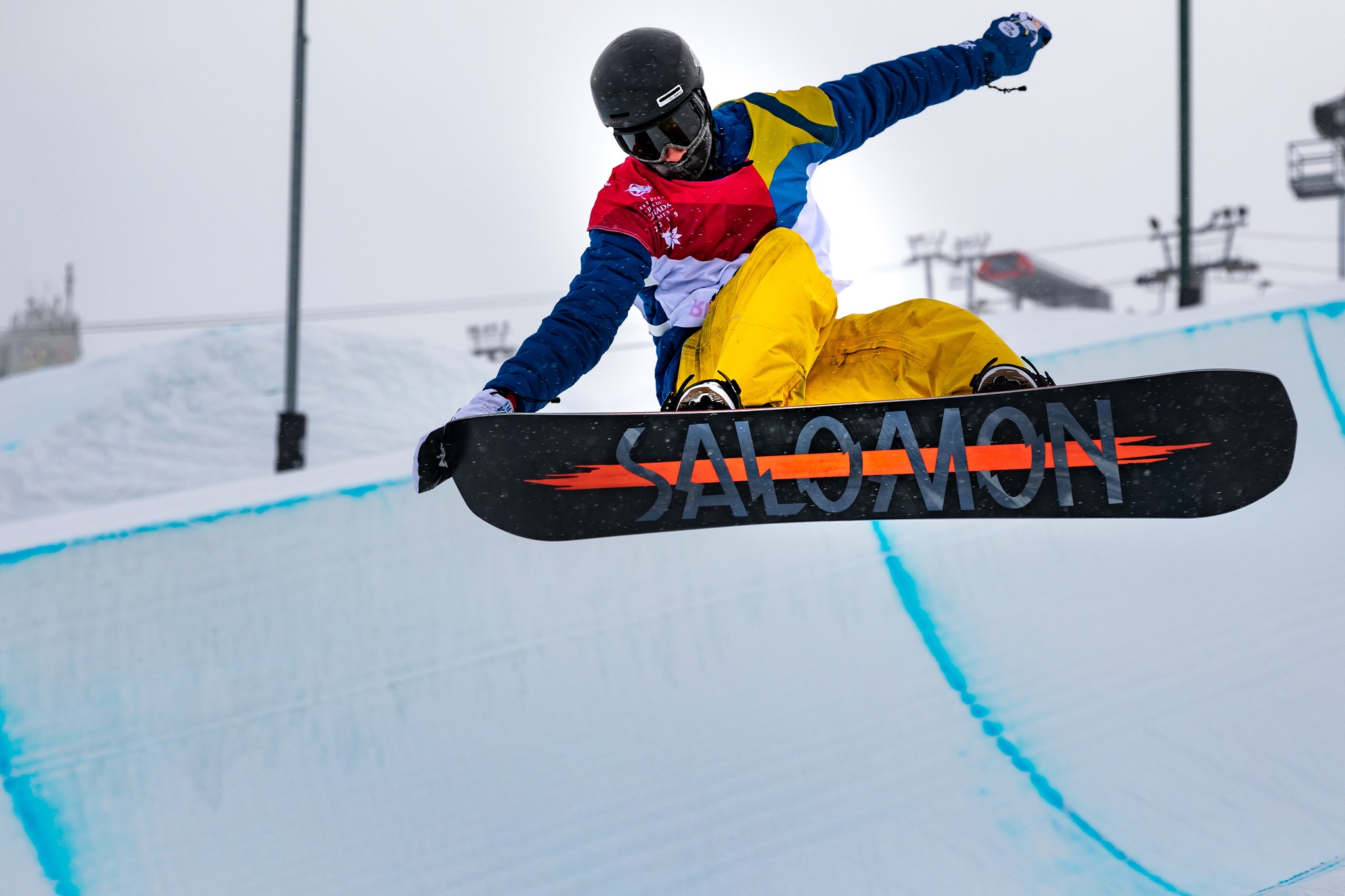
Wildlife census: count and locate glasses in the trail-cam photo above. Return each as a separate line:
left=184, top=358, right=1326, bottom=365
left=612, top=94, right=707, bottom=163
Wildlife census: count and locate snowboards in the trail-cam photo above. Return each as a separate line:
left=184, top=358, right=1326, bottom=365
left=442, top=368, right=1299, bottom=544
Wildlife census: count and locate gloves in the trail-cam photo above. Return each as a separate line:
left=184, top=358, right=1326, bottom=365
left=414, top=385, right=525, bottom=493
left=975, top=12, right=1051, bottom=89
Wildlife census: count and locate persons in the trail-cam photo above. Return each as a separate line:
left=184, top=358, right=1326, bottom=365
left=412, top=9, right=1055, bottom=494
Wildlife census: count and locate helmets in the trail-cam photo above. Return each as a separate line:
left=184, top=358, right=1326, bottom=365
left=589, top=27, right=712, bottom=180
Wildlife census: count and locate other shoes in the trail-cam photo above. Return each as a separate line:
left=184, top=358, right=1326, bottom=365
left=975, top=363, right=1040, bottom=394
left=676, top=379, right=739, bottom=411
